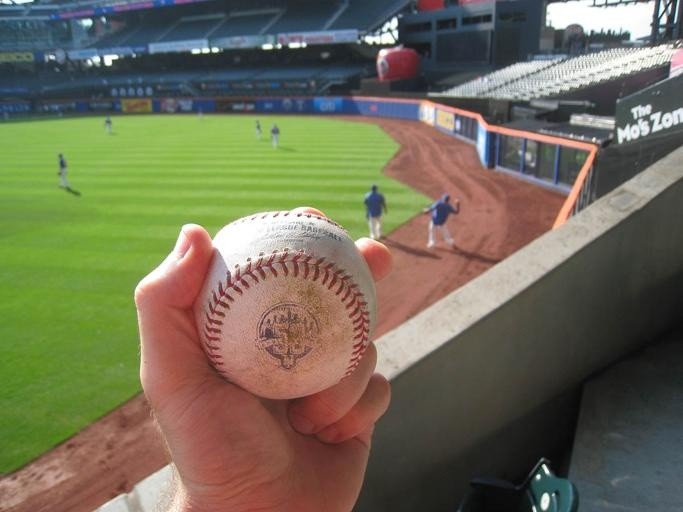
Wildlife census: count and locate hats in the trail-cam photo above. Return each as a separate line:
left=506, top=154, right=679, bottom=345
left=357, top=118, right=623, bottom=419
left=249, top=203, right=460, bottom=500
left=442, top=193, right=450, bottom=201
left=371, top=184, right=378, bottom=190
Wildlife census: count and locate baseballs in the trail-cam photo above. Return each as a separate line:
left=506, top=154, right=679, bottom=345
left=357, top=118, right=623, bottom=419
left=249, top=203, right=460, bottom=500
left=192, top=210, right=376, bottom=401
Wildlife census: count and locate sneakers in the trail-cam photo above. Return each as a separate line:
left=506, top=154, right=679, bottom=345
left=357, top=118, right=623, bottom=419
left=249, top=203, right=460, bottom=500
left=427, top=242, right=460, bottom=251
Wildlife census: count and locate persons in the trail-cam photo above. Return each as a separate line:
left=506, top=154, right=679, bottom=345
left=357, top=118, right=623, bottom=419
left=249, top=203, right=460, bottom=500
left=130, top=205, right=393, bottom=512
left=363, top=184, right=388, bottom=242
left=54, top=153, right=69, bottom=190
left=101, top=108, right=112, bottom=133
left=269, top=124, right=279, bottom=146
left=422, top=193, right=460, bottom=250
left=253, top=119, right=263, bottom=138
